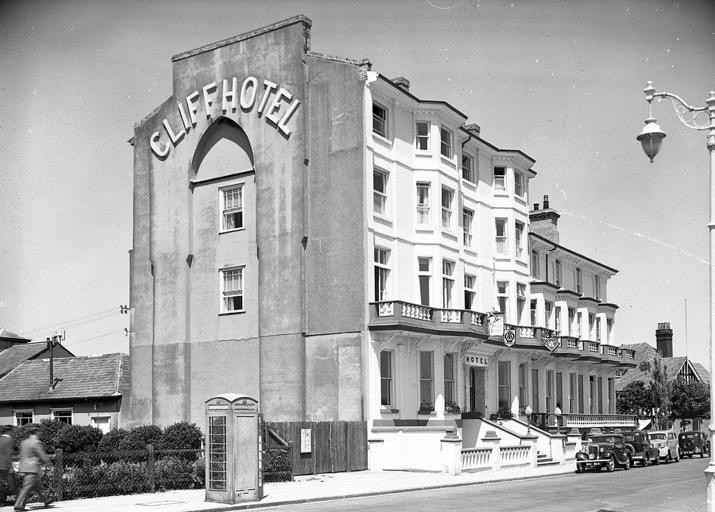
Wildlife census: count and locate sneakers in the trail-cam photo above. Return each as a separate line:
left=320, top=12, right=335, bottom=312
left=13, top=495, right=57, bottom=511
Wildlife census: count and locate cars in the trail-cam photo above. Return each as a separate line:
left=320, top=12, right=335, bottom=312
left=576, top=427, right=711, bottom=472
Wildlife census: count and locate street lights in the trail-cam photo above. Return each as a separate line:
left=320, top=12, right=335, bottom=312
left=554, top=406, right=563, bottom=435
left=524, top=406, right=534, bottom=438
left=636, top=77, right=715, bottom=512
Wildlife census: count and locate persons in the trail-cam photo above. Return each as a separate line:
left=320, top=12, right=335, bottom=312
left=12, top=424, right=54, bottom=512
left=0, top=425, right=17, bottom=507
